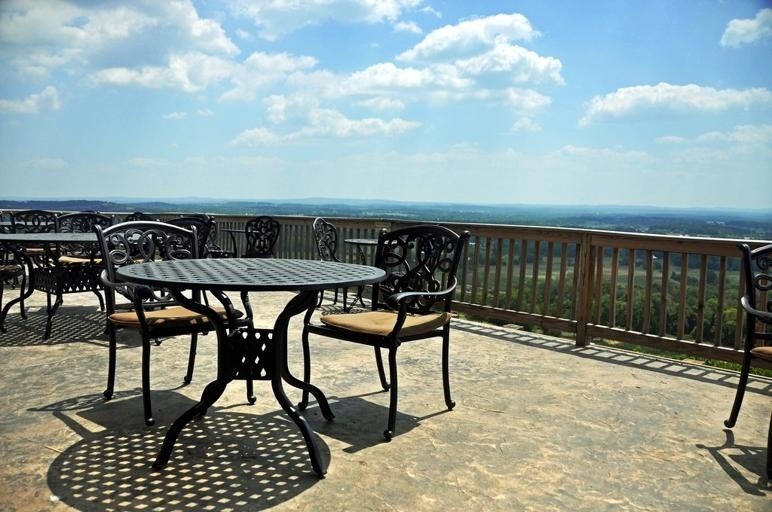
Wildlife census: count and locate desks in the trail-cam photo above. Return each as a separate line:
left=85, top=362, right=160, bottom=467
left=344, top=238, right=398, bottom=312
left=0, top=232, right=172, bottom=341
left=116, top=257, right=388, bottom=481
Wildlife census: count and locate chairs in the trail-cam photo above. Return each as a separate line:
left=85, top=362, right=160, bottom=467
left=241, top=216, right=282, bottom=257
left=54, top=213, right=115, bottom=263
left=298, top=224, right=470, bottom=440
left=8, top=213, right=56, bottom=252
left=0, top=211, right=24, bottom=290
left=203, top=216, right=238, bottom=257
left=94, top=221, right=244, bottom=425
left=312, top=217, right=366, bottom=312
left=724, top=242, right=772, bottom=481
left=155, top=216, right=215, bottom=256
left=119, top=212, right=151, bottom=222
